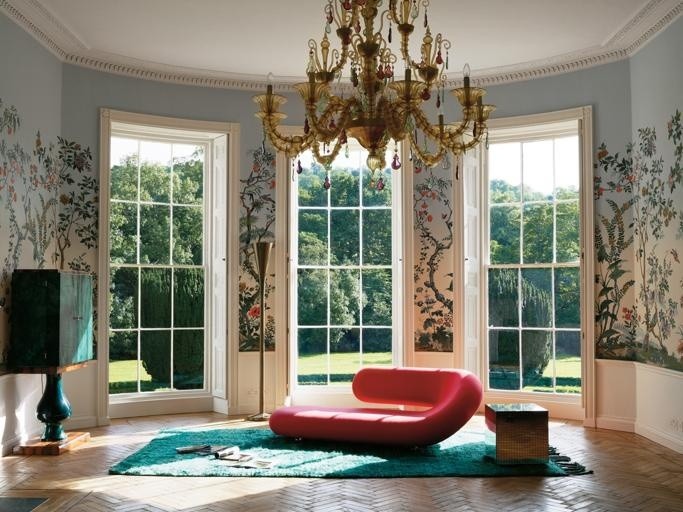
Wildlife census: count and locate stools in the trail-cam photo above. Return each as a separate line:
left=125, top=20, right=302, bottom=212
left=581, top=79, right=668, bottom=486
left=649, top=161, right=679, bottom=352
left=485, top=404, right=548, bottom=466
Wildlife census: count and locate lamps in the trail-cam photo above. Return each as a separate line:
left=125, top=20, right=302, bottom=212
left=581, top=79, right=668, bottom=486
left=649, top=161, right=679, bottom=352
left=250, top=0, right=498, bottom=190
left=247, top=241, right=273, bottom=421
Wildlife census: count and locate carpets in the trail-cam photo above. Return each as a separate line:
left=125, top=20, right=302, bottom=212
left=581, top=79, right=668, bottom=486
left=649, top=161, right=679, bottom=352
left=109, top=428, right=592, bottom=477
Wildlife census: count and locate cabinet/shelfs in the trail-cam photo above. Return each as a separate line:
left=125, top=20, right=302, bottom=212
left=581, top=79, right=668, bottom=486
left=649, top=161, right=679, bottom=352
left=9, top=269, right=93, bottom=368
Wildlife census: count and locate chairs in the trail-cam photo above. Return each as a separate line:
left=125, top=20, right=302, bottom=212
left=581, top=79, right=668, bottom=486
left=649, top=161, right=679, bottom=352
left=270, top=366, right=484, bottom=447
